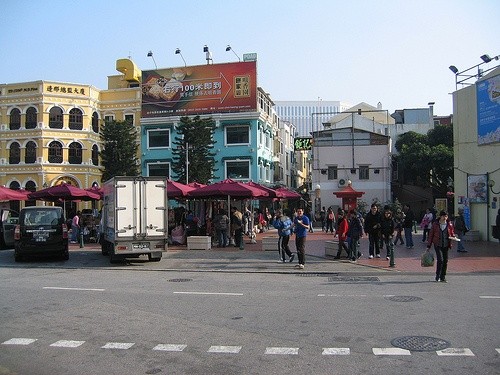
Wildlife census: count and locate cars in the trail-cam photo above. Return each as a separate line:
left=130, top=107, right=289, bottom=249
left=78, top=209, right=94, bottom=219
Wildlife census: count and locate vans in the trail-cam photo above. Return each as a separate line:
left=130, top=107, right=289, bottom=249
left=0, top=205, right=72, bottom=264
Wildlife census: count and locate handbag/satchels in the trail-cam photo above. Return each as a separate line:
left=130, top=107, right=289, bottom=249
left=464, top=225, right=469, bottom=232
left=421, top=248, right=434, bottom=267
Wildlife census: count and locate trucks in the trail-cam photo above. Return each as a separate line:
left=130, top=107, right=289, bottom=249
left=93, top=176, right=168, bottom=264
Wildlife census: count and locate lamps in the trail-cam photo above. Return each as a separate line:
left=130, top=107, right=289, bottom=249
left=148, top=51, right=157, bottom=70
left=480, top=54, right=491, bottom=62
left=204, top=45, right=213, bottom=64
left=449, top=66, right=458, bottom=73
left=175, top=47, right=186, bottom=67
left=226, top=45, right=240, bottom=62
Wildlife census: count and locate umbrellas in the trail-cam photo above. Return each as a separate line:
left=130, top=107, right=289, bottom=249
left=0, top=184, right=104, bottom=210
left=167, top=178, right=301, bottom=206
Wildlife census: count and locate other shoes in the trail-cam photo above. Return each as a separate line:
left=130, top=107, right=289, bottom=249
left=70, top=241, right=79, bottom=243
left=296, top=263, right=305, bottom=269
left=435, top=277, right=449, bottom=283
left=376, top=253, right=380, bottom=258
left=289, top=253, right=294, bottom=262
left=368, top=254, right=373, bottom=258
left=277, top=258, right=287, bottom=263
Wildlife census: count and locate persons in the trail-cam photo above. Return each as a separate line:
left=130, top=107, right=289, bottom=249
left=272, top=209, right=294, bottom=263
left=334, top=209, right=349, bottom=259
left=426, top=210, right=457, bottom=283
left=346, top=209, right=365, bottom=261
left=273, top=206, right=283, bottom=221
left=292, top=208, right=297, bottom=234
left=70, top=210, right=99, bottom=244
left=364, top=203, right=381, bottom=259
left=282, top=208, right=310, bottom=269
left=308, top=206, right=368, bottom=235
left=382, top=204, right=500, bottom=260
left=179, top=207, right=271, bottom=248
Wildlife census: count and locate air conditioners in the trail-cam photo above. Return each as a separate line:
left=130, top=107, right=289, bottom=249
left=339, top=179, right=348, bottom=187
